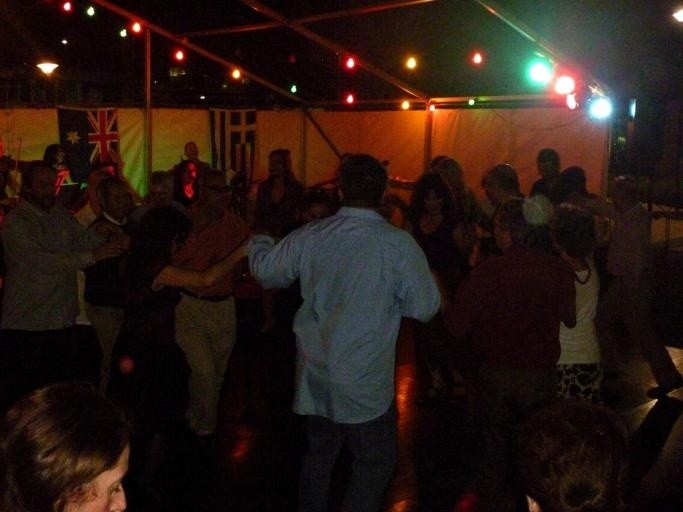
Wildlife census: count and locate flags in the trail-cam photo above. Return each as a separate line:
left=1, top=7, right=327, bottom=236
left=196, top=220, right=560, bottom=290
left=206, top=109, right=258, bottom=186
left=55, top=105, right=121, bottom=184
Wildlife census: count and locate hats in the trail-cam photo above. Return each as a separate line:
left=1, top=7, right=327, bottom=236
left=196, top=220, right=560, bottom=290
left=560, top=167, right=584, bottom=181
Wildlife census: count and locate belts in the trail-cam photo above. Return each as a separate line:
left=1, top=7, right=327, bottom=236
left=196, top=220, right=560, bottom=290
left=182, top=290, right=233, bottom=301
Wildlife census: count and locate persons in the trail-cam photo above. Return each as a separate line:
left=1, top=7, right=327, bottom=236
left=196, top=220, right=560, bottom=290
left=0, top=138, right=683, bottom=512
